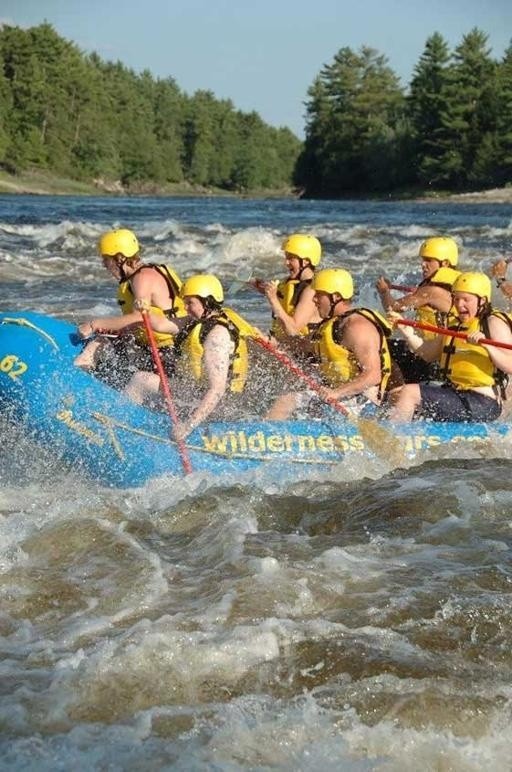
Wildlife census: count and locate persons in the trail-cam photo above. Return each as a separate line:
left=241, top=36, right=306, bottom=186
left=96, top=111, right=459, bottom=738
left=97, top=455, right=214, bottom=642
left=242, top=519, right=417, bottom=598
left=488, top=257, right=511, bottom=301
left=252, top=233, right=323, bottom=342
left=383, top=271, right=512, bottom=430
left=124, top=272, right=250, bottom=443
left=69, top=230, right=186, bottom=392
left=261, top=262, right=391, bottom=422
left=375, top=235, right=466, bottom=348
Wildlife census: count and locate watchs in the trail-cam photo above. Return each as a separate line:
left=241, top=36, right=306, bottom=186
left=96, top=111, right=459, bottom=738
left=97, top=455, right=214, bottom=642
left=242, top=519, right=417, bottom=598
left=494, top=277, right=507, bottom=288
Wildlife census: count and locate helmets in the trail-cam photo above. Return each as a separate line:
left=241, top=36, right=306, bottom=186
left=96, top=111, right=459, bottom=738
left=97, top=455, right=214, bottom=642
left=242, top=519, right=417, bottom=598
left=419, top=237, right=458, bottom=266
left=452, top=271, right=491, bottom=303
left=180, top=274, right=224, bottom=302
left=99, top=229, right=140, bottom=258
left=280, top=234, right=322, bottom=267
left=311, top=268, right=353, bottom=299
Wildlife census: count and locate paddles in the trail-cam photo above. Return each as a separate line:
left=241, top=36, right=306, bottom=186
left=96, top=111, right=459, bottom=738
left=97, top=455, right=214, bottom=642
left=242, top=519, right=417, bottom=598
left=254, top=337, right=408, bottom=468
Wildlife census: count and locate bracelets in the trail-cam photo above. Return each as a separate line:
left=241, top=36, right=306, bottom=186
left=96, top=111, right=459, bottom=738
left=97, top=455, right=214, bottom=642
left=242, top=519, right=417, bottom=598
left=185, top=415, right=198, bottom=429
left=89, top=319, right=97, bottom=331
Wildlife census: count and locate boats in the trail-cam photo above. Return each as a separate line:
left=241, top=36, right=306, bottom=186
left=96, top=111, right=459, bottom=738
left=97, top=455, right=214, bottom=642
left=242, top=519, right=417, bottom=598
left=0, top=308, right=509, bottom=493
